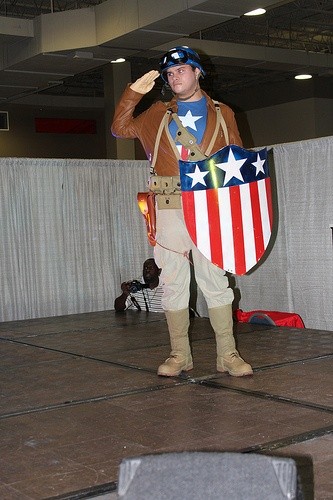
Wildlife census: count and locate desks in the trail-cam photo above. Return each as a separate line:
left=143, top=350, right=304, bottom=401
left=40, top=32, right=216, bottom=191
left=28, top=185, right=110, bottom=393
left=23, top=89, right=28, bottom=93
left=236, top=309, right=306, bottom=329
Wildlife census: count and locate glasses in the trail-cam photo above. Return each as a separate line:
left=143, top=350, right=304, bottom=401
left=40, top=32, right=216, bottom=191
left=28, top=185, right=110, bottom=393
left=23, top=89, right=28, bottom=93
left=159, top=49, right=203, bottom=68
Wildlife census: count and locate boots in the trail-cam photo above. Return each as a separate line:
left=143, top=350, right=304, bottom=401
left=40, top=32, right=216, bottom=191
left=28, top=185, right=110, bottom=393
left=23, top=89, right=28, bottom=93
left=206, top=304, right=253, bottom=377
left=157, top=309, right=194, bottom=375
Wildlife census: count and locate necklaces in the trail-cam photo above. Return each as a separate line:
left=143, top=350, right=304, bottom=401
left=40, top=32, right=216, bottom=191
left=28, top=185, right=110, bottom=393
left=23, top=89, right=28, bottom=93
left=145, top=287, right=157, bottom=309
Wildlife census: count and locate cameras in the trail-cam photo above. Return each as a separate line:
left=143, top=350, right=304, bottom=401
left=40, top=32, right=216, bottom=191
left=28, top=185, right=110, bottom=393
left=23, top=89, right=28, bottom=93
left=130, top=280, right=141, bottom=293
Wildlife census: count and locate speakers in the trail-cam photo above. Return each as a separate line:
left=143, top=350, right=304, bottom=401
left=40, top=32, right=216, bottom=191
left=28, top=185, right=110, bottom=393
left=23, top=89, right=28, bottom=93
left=118, top=452, right=305, bottom=500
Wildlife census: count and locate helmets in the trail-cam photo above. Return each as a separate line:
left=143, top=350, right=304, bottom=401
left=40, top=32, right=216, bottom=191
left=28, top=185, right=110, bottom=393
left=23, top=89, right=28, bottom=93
left=160, top=46, right=205, bottom=83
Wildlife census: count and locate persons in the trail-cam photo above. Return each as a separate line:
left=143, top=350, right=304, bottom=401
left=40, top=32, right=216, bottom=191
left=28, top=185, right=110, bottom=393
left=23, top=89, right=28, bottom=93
left=114, top=258, right=166, bottom=313
left=110, top=45, right=255, bottom=379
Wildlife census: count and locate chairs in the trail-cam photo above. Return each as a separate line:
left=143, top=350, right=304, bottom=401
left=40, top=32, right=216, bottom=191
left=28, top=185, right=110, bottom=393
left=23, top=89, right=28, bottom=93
left=249, top=313, right=276, bottom=327
